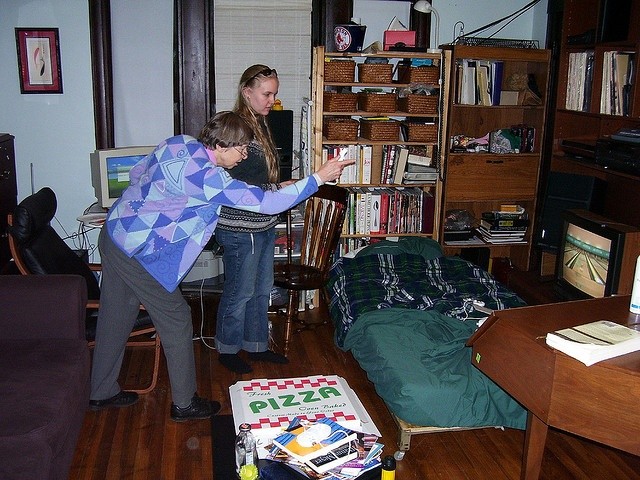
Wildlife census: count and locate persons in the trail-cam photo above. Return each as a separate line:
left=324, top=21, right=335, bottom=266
left=214, top=64, right=297, bottom=374
left=89, top=110, right=356, bottom=420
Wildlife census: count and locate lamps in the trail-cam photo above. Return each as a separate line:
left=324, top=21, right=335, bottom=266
left=414, top=0, right=442, bottom=54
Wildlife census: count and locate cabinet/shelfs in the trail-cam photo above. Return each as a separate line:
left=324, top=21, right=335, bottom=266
left=509, top=1, right=640, bottom=304
left=309, top=44, right=451, bottom=299
left=436, top=44, right=551, bottom=287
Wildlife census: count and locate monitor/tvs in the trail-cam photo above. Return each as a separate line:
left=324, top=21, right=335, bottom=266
left=88, top=147, right=159, bottom=208
left=553, top=211, right=622, bottom=299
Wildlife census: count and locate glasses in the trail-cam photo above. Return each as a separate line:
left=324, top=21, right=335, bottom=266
left=235, top=148, right=251, bottom=159
left=245, top=68, right=278, bottom=85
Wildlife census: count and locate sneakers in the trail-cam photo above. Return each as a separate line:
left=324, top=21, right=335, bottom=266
left=88, top=391, right=139, bottom=409
left=170, top=396, right=222, bottom=423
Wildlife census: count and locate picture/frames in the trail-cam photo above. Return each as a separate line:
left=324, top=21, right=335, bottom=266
left=14, top=28, right=62, bottom=95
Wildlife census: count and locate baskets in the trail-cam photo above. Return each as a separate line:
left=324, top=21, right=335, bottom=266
left=361, top=92, right=396, bottom=113
left=363, top=118, right=401, bottom=141
left=358, top=63, right=393, bottom=83
left=323, top=92, right=358, bottom=112
left=325, top=118, right=360, bottom=140
left=403, top=121, right=439, bottom=142
left=324, top=59, right=356, bottom=83
left=403, top=94, right=438, bottom=114
left=397, top=66, right=439, bottom=84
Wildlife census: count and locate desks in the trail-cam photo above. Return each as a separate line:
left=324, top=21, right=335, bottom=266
left=464, top=295, right=640, bottom=480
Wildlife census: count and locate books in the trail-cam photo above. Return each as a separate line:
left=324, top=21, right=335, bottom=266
left=455, top=58, right=503, bottom=105
left=323, top=145, right=439, bottom=184
left=342, top=187, right=434, bottom=234
left=268, top=419, right=385, bottom=480
left=565, top=53, right=595, bottom=112
left=600, top=50, right=635, bottom=117
left=479, top=210, right=528, bottom=243
left=327, top=237, right=371, bottom=266
left=545, top=320, right=640, bottom=368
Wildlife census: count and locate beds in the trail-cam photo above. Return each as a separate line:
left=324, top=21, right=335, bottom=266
left=325, top=237, right=528, bottom=450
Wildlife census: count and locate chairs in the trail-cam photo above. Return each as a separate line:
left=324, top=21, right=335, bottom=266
left=273, top=177, right=348, bottom=357
left=9, top=187, right=160, bottom=395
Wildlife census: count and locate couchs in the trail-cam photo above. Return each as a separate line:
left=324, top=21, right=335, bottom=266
left=0, top=275, right=89, bottom=480
